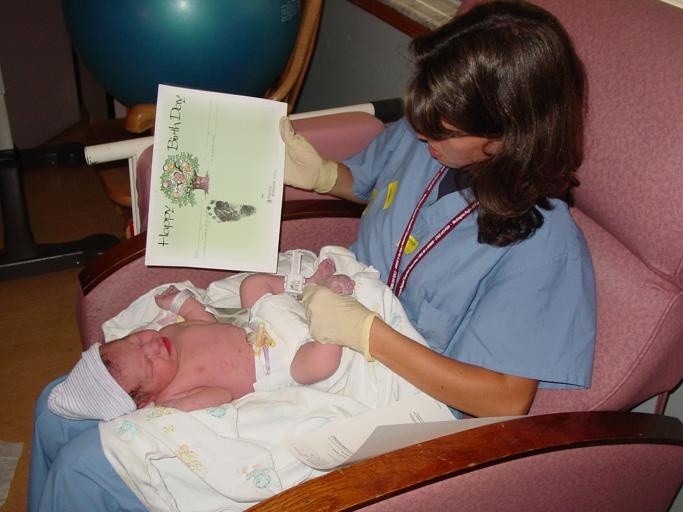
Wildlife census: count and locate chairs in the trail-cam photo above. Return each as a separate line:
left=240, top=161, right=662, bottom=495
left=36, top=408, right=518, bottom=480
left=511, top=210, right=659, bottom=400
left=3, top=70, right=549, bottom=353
left=74, top=2, right=682, bottom=511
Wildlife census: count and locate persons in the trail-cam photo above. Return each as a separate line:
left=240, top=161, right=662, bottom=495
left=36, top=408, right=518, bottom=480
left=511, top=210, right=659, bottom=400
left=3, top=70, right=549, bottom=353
left=47, top=255, right=356, bottom=424
left=27, top=0, right=596, bottom=512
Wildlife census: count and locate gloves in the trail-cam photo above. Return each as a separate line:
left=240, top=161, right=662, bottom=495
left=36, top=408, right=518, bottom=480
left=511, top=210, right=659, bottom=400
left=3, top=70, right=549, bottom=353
left=299, top=282, right=382, bottom=363
left=278, top=115, right=339, bottom=195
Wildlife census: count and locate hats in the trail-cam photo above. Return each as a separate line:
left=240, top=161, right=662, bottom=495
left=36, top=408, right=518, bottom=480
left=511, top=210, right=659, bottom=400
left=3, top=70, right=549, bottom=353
left=46, top=342, right=137, bottom=423
